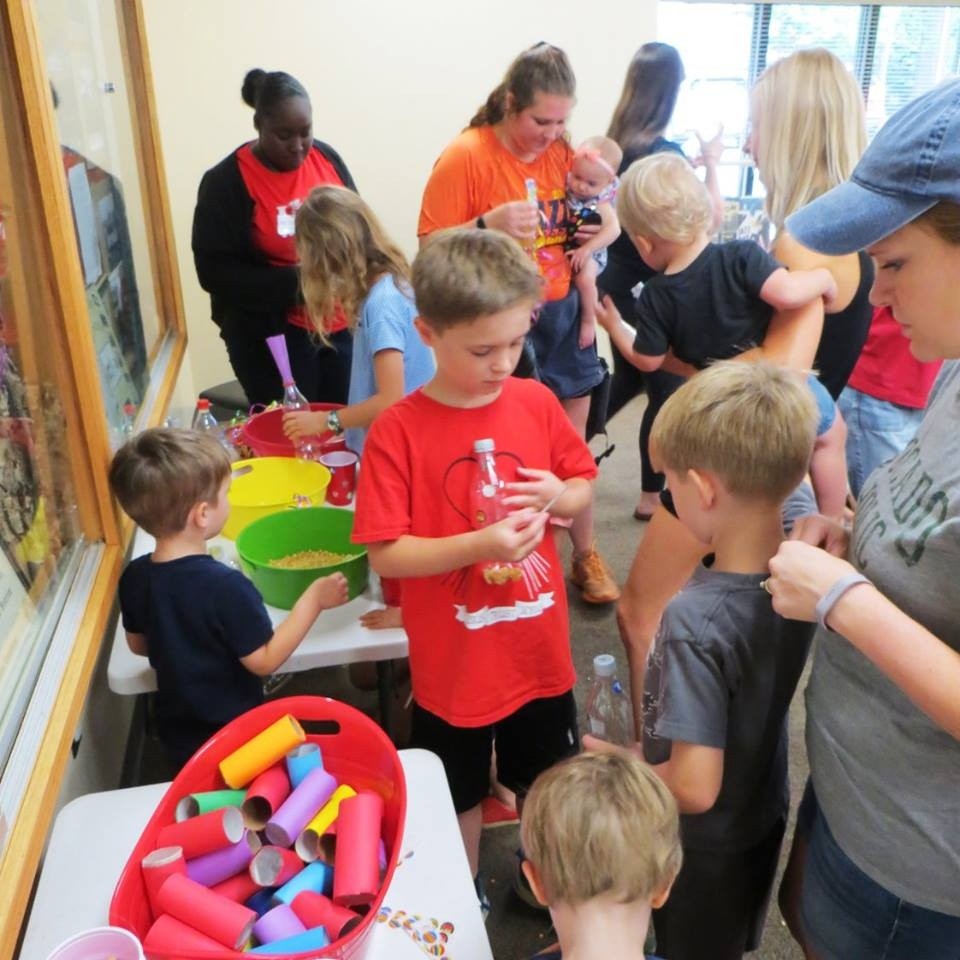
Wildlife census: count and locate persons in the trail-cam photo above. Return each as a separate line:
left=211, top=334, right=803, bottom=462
left=520, top=750, right=683, bottom=960
left=582, top=346, right=821, bottom=960
left=192, top=71, right=362, bottom=412
left=594, top=47, right=876, bottom=749
left=417, top=37, right=622, bottom=605
left=758, top=75, right=960, bottom=960
left=351, top=224, right=601, bottom=881
left=282, top=185, right=437, bottom=463
left=108, top=432, right=350, bottom=777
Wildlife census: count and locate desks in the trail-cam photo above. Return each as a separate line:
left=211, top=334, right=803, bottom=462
left=106, top=426, right=414, bottom=753
left=12, top=749, right=494, bottom=960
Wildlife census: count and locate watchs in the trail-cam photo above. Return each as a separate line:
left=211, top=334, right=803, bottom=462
left=327, top=410, right=343, bottom=436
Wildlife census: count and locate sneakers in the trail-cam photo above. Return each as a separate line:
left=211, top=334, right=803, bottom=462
left=570, top=550, right=623, bottom=603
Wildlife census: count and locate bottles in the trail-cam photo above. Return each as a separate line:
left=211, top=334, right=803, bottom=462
left=589, top=654, right=638, bottom=749
left=193, top=399, right=217, bottom=433
left=470, top=439, right=522, bottom=583
left=282, top=378, right=322, bottom=461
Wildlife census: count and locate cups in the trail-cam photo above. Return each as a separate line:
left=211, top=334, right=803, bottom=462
left=42, top=925, right=148, bottom=958
left=319, top=451, right=356, bottom=507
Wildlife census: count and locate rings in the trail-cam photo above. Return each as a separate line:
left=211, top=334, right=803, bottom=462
left=759, top=580, right=772, bottom=595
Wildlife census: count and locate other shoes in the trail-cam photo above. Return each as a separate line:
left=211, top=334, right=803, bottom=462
left=483, top=799, right=518, bottom=827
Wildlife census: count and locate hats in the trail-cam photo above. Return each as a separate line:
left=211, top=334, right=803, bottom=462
left=784, top=79, right=960, bottom=256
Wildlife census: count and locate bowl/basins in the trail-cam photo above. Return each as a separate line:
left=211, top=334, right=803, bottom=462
left=220, top=457, right=330, bottom=543
left=235, top=507, right=371, bottom=611
left=110, top=695, right=407, bottom=960
left=242, top=403, right=351, bottom=458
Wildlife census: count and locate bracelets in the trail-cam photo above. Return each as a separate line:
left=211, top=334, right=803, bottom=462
left=476, top=215, right=486, bottom=229
left=816, top=573, right=873, bottom=635
